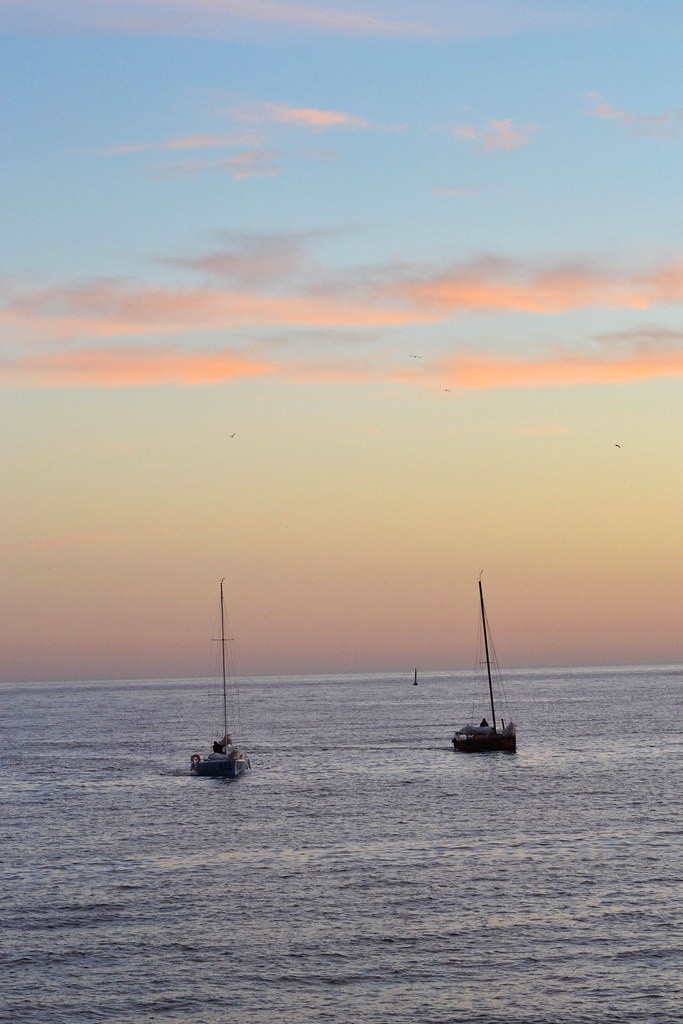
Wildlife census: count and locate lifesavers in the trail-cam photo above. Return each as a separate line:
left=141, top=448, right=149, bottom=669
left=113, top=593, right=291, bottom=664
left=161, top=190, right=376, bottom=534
left=190, top=754, right=201, bottom=763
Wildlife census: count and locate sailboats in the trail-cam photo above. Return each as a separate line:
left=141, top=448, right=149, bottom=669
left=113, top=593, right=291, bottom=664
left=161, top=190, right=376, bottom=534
left=189, top=575, right=251, bottom=777
left=450, top=580, right=517, bottom=751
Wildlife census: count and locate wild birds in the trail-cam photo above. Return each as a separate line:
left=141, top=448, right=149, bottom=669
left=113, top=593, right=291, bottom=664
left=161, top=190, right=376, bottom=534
left=229, top=432, right=236, bottom=438
left=444, top=389, right=451, bottom=394
left=614, top=444, right=621, bottom=450
left=407, top=351, right=424, bottom=359
